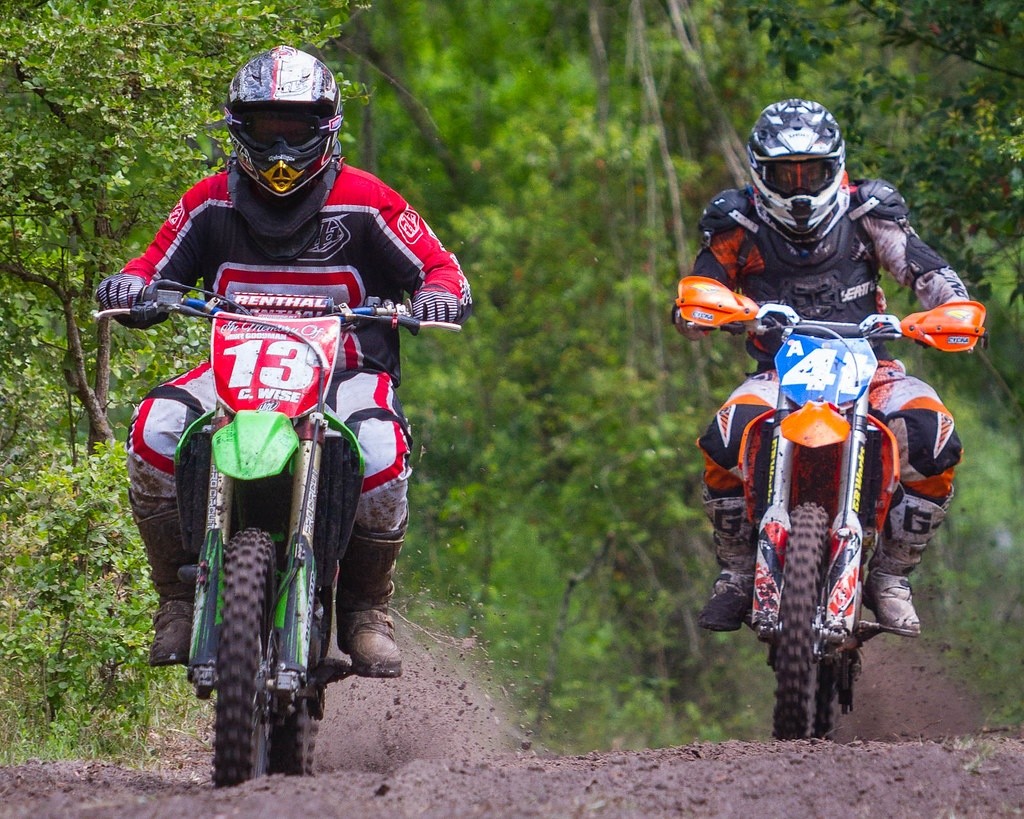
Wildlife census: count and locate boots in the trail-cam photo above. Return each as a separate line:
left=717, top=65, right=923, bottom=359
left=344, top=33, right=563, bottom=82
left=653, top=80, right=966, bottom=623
left=336, top=506, right=411, bottom=677
left=697, top=482, right=761, bottom=631
left=863, top=486, right=955, bottom=637
left=128, top=512, right=195, bottom=666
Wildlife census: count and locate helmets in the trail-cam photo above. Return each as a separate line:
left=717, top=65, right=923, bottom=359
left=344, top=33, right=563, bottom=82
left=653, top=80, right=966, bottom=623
left=747, top=98, right=847, bottom=235
left=224, top=45, right=345, bottom=197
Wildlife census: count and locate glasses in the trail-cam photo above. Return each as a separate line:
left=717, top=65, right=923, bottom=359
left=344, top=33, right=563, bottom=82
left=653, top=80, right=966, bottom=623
left=239, top=113, right=323, bottom=152
left=757, top=161, right=840, bottom=195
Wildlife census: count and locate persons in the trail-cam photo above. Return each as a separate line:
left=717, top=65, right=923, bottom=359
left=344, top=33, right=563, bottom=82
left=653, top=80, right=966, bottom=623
left=672, top=97, right=972, bottom=637
left=92, top=47, right=473, bottom=677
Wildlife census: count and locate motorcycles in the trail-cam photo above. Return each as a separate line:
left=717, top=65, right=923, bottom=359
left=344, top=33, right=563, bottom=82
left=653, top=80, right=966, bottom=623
left=92, top=278, right=464, bottom=792
left=672, top=276, right=988, bottom=739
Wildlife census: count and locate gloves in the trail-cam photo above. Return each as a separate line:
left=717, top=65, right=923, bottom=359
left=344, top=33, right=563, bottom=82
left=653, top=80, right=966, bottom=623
left=96, top=273, right=147, bottom=309
left=413, top=287, right=464, bottom=323
